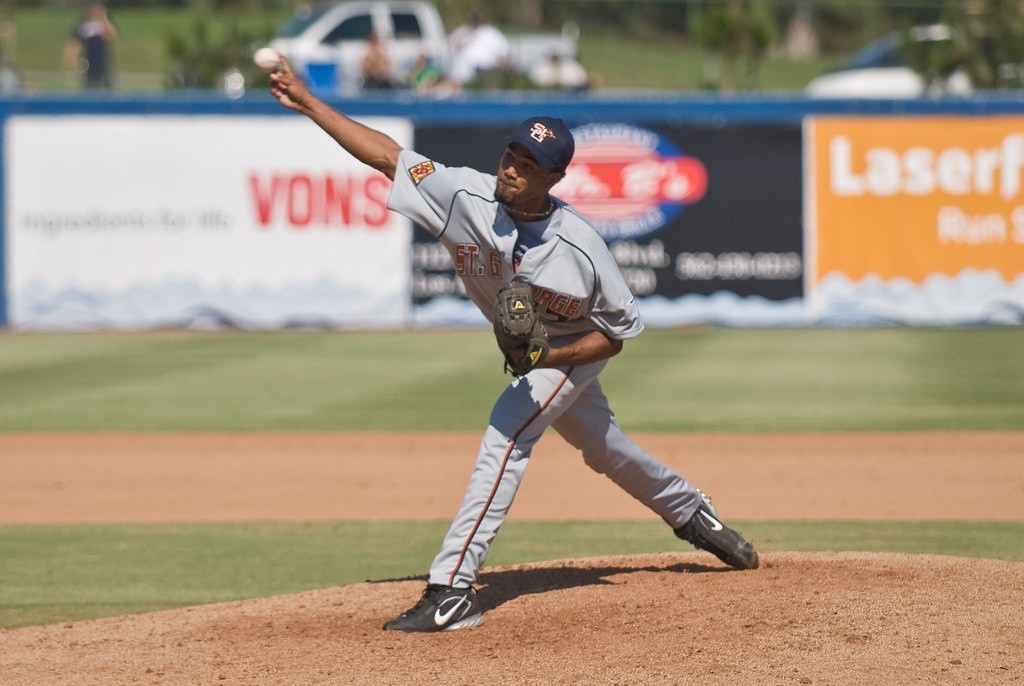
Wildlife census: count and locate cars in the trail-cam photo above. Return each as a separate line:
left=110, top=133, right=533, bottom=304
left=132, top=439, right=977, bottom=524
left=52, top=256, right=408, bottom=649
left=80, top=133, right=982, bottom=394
left=800, top=23, right=1024, bottom=103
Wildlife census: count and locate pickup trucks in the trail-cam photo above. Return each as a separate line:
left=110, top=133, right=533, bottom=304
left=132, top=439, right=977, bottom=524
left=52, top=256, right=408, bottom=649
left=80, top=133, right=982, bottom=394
left=269, top=0, right=582, bottom=89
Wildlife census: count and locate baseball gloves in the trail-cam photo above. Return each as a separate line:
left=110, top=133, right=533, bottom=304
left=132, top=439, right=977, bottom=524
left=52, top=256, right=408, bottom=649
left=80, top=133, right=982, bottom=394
left=494, top=275, right=550, bottom=384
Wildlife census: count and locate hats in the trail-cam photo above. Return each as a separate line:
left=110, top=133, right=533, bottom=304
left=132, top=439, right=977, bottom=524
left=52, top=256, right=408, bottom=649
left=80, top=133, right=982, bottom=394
left=503, top=116, right=575, bottom=172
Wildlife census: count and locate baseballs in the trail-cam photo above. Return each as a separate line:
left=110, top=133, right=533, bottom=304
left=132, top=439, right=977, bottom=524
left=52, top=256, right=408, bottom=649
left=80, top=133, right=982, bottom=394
left=253, top=47, right=279, bottom=74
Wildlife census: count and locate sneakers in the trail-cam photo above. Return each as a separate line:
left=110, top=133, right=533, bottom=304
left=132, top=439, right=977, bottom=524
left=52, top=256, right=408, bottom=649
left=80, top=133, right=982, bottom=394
left=383, top=579, right=484, bottom=635
left=674, top=489, right=760, bottom=570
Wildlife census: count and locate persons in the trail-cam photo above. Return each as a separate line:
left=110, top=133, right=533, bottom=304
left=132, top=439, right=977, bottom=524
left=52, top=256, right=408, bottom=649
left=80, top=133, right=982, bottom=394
left=271, top=55, right=759, bottom=634
left=66, top=0, right=118, bottom=90
left=361, top=37, right=439, bottom=87
